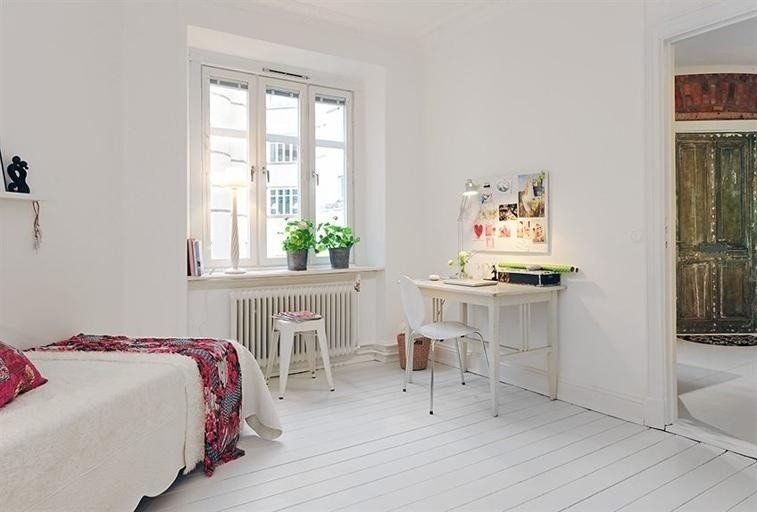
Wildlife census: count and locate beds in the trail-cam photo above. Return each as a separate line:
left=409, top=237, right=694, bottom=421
left=0, top=332, right=284, bottom=512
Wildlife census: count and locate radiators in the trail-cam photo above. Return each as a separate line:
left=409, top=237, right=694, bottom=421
left=228, top=282, right=361, bottom=376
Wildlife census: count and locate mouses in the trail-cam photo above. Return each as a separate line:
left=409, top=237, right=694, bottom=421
left=429, top=274, right=440, bottom=281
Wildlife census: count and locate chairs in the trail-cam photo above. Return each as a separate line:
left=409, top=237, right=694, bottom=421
left=400, top=275, right=491, bottom=416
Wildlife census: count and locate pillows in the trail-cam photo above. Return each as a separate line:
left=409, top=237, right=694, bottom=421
left=0, top=339, right=48, bottom=409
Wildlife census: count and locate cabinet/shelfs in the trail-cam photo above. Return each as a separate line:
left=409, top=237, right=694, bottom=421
left=675, top=132, right=757, bottom=347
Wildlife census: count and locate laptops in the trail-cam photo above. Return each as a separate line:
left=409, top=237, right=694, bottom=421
left=444, top=279, right=498, bottom=287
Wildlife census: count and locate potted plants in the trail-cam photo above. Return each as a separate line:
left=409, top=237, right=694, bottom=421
left=314, top=216, right=360, bottom=268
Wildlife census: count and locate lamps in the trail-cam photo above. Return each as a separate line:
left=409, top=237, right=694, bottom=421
left=443, top=178, right=479, bottom=278
left=211, top=168, right=255, bottom=275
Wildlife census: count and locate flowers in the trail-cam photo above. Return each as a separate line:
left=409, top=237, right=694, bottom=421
left=276, top=215, right=314, bottom=251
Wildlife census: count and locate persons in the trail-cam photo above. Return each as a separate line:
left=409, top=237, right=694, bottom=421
left=498, top=205, right=511, bottom=221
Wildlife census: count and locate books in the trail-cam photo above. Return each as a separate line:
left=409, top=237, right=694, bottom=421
left=271, top=310, right=322, bottom=322
left=445, top=279, right=499, bottom=287
left=188, top=239, right=204, bottom=276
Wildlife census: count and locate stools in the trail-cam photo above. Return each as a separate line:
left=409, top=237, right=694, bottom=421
left=265, top=314, right=335, bottom=401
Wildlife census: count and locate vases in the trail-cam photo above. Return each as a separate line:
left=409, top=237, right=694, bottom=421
left=285, top=248, right=308, bottom=271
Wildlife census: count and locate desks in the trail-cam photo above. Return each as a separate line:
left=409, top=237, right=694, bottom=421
left=414, top=279, right=567, bottom=417
